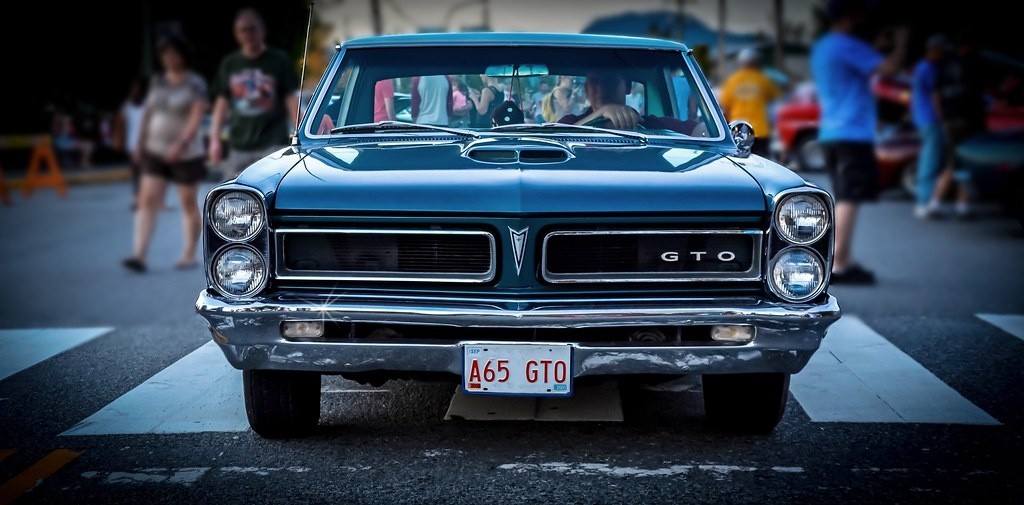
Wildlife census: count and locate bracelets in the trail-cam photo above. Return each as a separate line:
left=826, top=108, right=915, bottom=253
left=571, top=94, right=576, bottom=98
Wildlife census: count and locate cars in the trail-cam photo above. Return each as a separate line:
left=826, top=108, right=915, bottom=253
left=775, top=71, right=1024, bottom=214
left=195, top=31, right=843, bottom=436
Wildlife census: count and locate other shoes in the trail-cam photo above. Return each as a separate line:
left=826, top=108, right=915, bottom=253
left=122, top=258, right=147, bottom=273
left=830, top=263, right=875, bottom=285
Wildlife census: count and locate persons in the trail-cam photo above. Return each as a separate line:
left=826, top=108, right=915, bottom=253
left=557, top=69, right=711, bottom=138
left=55, top=0, right=1024, bottom=285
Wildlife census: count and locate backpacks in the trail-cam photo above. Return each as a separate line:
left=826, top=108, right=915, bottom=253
left=541, top=88, right=569, bottom=124
